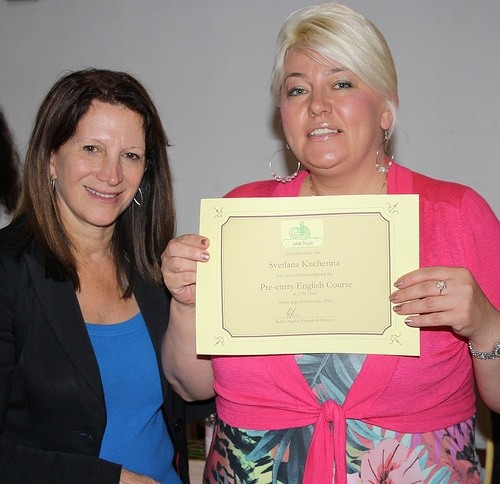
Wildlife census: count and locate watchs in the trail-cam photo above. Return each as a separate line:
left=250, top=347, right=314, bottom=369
left=469, top=340, right=500, bottom=359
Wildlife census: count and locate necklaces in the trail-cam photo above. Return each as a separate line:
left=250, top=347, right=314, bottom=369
left=309, top=173, right=387, bottom=195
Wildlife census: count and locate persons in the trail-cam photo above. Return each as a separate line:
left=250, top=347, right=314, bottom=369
left=0, top=66, right=191, bottom=484
left=160, top=0, right=500, bottom=483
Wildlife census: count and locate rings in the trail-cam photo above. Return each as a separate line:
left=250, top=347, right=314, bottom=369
left=437, top=280, right=448, bottom=295
